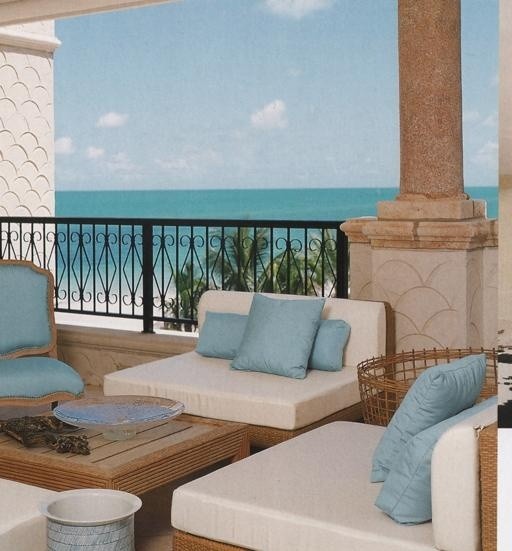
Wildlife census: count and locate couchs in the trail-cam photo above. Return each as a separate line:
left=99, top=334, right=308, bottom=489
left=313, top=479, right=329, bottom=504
left=102, top=289, right=396, bottom=450
left=0, top=477, right=60, bottom=551
left=170, top=395, right=511, bottom=550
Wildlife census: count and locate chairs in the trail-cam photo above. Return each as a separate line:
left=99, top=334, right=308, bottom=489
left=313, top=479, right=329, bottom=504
left=0, top=259, right=87, bottom=419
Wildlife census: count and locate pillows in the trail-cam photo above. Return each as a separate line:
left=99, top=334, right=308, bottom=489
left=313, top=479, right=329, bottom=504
left=196, top=311, right=351, bottom=372
left=231, top=292, right=325, bottom=379
left=375, top=395, right=497, bottom=525
left=368, top=353, right=486, bottom=484
